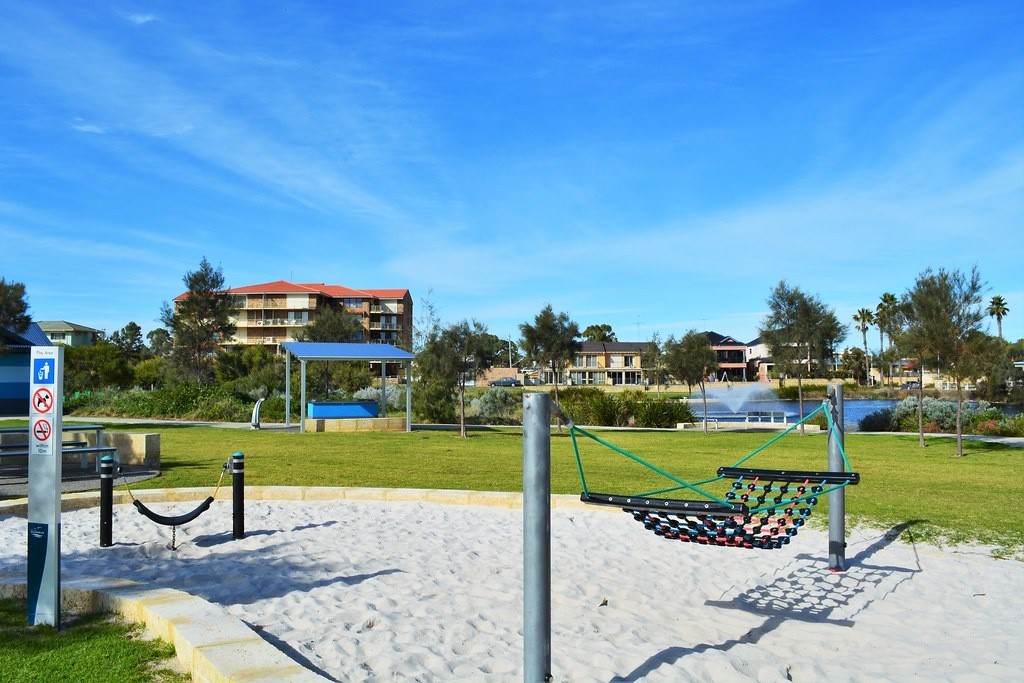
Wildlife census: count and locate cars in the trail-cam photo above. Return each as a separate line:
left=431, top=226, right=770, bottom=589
left=898, top=380, right=919, bottom=390
left=487, top=377, right=521, bottom=387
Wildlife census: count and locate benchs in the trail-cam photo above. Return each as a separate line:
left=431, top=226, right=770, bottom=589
left=0, top=445, right=117, bottom=477
left=0, top=441, right=88, bottom=468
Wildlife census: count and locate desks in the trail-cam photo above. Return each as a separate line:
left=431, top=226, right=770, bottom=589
left=0, top=424, right=105, bottom=473
